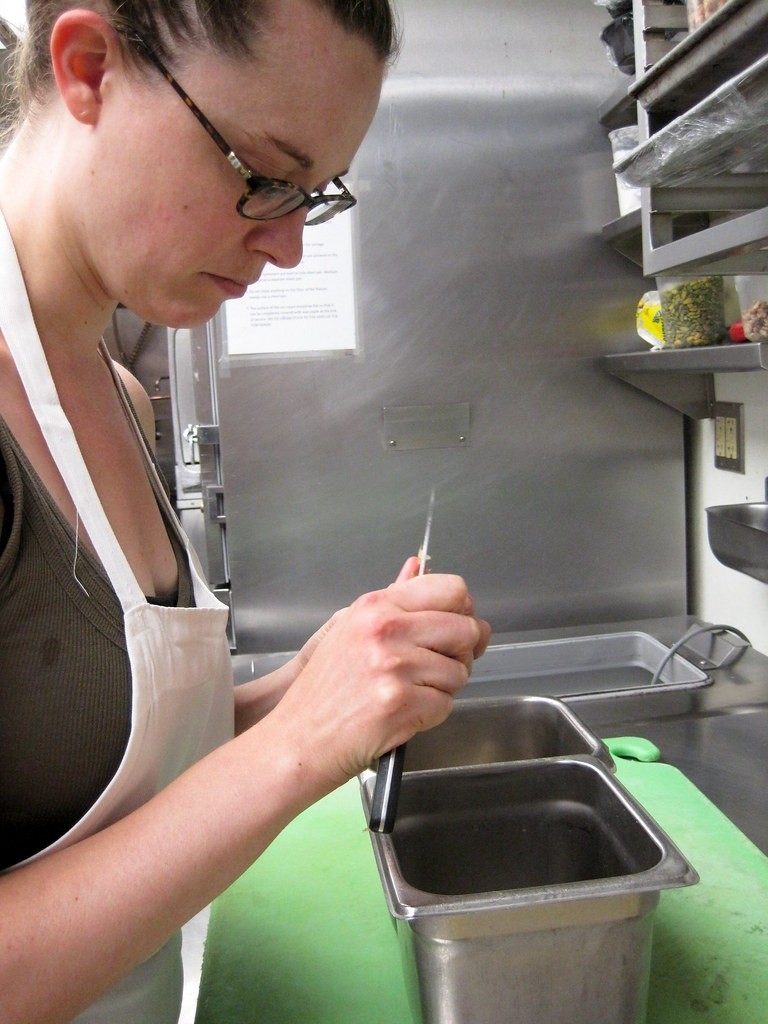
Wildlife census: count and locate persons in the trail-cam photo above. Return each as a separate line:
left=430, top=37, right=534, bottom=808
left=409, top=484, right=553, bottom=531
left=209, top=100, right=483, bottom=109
left=0, top=0, right=489, bottom=1023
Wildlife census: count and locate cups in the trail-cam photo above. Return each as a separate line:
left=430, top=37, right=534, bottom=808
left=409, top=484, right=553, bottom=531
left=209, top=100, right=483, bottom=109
left=733, top=275, right=768, bottom=343
left=607, top=125, right=642, bottom=217
left=654, top=274, right=728, bottom=348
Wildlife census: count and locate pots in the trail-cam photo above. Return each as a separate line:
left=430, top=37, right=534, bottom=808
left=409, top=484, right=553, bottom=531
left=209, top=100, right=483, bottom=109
left=600, top=11, right=634, bottom=75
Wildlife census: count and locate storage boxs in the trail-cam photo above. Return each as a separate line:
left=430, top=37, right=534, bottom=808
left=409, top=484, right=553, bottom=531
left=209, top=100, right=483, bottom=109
left=365, top=754, right=702, bottom=1024
left=351, top=693, right=616, bottom=784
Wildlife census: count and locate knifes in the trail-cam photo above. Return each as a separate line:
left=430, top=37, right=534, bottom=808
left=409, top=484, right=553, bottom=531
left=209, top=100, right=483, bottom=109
left=368, top=487, right=438, bottom=835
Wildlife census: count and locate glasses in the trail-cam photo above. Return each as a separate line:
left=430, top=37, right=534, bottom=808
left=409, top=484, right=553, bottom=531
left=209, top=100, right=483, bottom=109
left=115, top=23, right=357, bottom=227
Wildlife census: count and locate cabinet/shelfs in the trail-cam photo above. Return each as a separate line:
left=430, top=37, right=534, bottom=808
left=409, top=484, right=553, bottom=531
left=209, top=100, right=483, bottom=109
left=601, top=0, right=768, bottom=423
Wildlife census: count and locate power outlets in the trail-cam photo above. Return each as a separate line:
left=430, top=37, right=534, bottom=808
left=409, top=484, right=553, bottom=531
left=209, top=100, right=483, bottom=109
left=713, top=401, right=746, bottom=475
